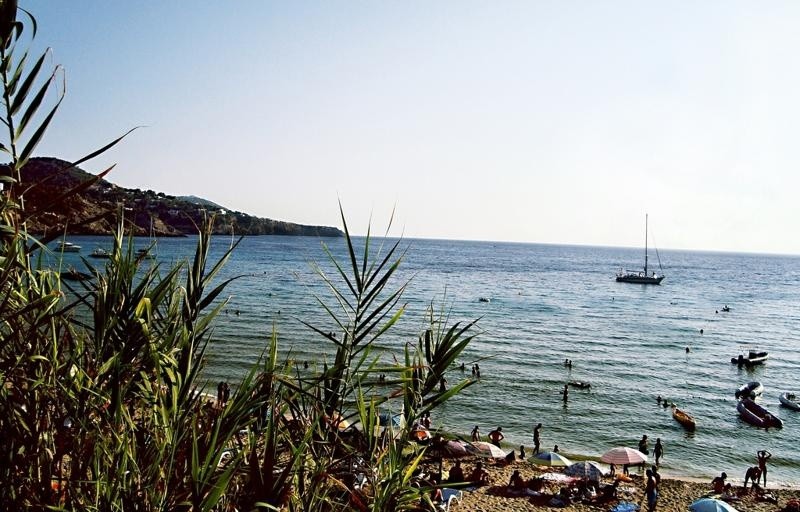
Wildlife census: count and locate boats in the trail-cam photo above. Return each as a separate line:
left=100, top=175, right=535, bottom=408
left=670, top=346, right=800, bottom=433
left=52, top=240, right=159, bottom=284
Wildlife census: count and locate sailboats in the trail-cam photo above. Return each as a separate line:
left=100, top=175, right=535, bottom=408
left=614, top=211, right=668, bottom=286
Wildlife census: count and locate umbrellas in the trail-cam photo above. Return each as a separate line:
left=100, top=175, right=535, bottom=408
left=314, top=410, right=351, bottom=429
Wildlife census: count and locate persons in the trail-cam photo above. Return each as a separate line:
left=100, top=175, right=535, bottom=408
left=472, top=366, right=476, bottom=376
left=561, top=384, right=568, bottom=403
left=222, top=382, right=230, bottom=408
left=439, top=376, right=447, bottom=392
left=475, top=363, right=480, bottom=376
left=217, top=381, right=223, bottom=408
left=355, top=411, right=772, bottom=511
